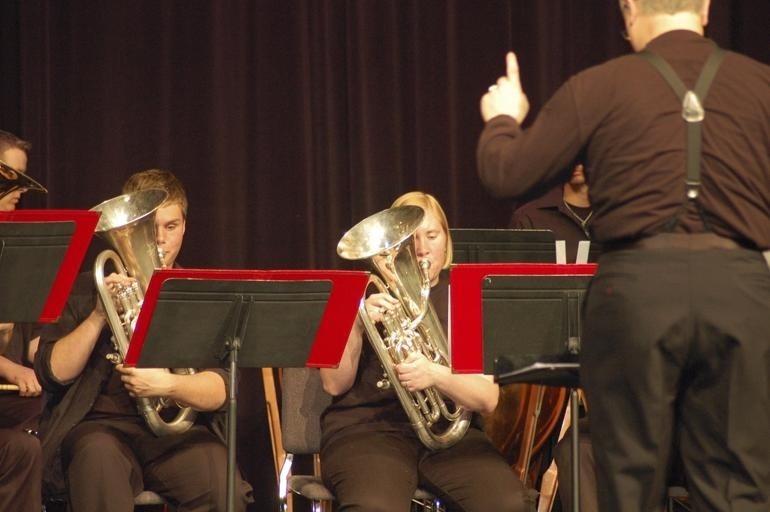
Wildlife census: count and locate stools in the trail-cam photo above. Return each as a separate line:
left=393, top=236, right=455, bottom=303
left=134, top=475, right=172, bottom=511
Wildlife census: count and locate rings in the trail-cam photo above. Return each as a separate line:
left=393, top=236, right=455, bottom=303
left=406, top=381, right=409, bottom=388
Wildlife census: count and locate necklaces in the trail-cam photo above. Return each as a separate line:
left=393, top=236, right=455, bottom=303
left=564, top=200, right=593, bottom=226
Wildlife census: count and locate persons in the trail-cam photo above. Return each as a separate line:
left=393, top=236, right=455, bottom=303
left=509, top=161, right=598, bottom=511
left=318, top=190, right=535, bottom=511
left=32, top=167, right=247, bottom=512
left=0, top=128, right=51, bottom=512
left=476, top=1, right=770, bottom=512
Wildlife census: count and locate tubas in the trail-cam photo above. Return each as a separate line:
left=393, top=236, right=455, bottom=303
left=337, top=205, right=473, bottom=451
left=87, top=188, right=201, bottom=437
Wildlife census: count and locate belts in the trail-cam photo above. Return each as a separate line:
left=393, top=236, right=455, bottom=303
left=629, top=230, right=740, bottom=252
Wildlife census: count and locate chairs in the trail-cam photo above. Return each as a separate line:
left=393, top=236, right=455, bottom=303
left=279, top=367, right=446, bottom=512
